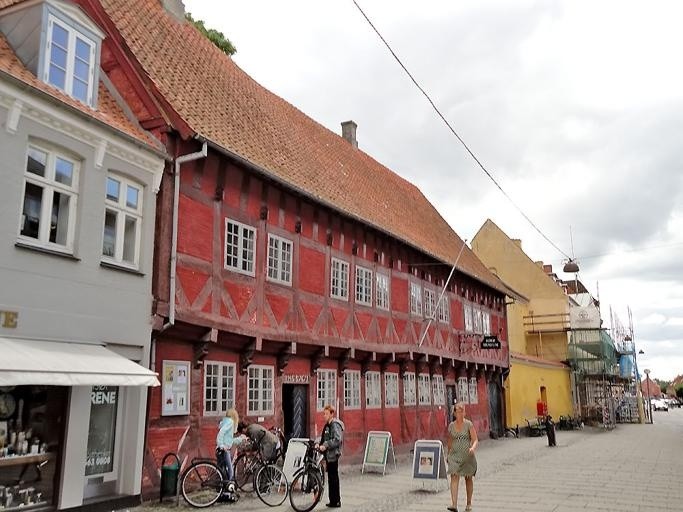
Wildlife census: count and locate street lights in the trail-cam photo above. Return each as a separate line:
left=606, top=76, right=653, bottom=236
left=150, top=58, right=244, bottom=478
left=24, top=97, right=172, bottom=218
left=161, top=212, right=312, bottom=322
left=644, top=369, right=652, bottom=423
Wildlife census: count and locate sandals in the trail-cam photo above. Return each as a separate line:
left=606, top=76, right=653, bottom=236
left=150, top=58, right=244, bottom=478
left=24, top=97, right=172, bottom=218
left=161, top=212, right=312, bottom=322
left=448, top=506, right=472, bottom=511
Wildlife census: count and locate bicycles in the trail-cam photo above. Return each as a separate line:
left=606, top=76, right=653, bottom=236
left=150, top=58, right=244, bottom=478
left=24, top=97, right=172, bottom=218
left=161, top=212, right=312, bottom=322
left=181, top=426, right=289, bottom=508
left=289, top=439, right=325, bottom=512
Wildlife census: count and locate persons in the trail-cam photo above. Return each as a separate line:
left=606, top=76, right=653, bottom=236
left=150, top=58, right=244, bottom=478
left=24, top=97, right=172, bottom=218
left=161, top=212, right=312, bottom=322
left=314, top=405, right=345, bottom=508
left=545, top=416, right=557, bottom=447
left=444, top=403, right=479, bottom=512
left=237, top=423, right=281, bottom=497
left=213, top=410, right=245, bottom=505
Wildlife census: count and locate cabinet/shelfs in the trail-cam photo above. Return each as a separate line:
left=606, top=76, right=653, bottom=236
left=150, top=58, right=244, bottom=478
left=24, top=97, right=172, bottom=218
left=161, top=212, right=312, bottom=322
left=0, top=452, right=57, bottom=512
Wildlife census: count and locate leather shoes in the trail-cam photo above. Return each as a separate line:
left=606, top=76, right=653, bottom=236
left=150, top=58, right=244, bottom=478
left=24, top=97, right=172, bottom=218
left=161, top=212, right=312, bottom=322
left=326, top=501, right=341, bottom=507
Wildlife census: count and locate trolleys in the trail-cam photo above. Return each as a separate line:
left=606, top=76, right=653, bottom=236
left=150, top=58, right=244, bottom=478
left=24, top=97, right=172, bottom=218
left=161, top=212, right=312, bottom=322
left=560, top=415, right=578, bottom=430
left=525, top=418, right=555, bottom=437
